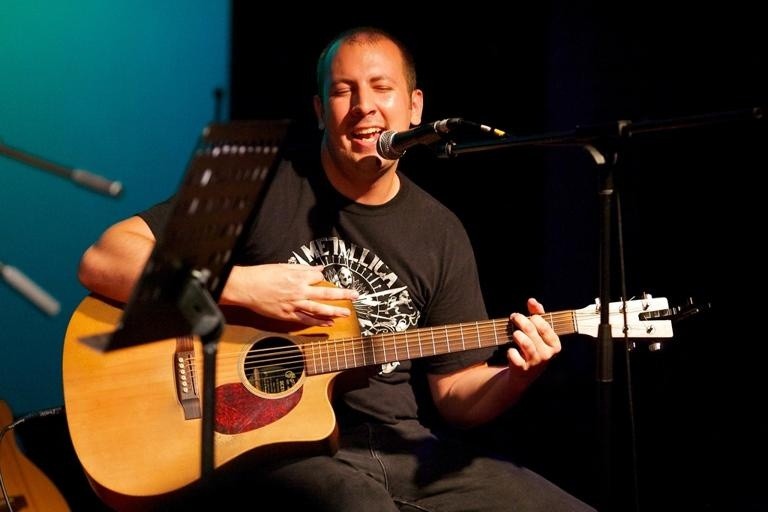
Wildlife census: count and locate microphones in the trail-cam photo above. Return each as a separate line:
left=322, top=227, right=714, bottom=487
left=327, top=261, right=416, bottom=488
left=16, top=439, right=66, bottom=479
left=376, top=117, right=462, bottom=161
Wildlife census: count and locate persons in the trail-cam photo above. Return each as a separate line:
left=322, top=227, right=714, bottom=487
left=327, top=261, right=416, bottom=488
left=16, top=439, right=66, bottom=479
left=79, top=29, right=597, bottom=512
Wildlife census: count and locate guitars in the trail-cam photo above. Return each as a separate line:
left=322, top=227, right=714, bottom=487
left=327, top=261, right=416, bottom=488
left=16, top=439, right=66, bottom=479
left=0, top=401, right=68, bottom=511
left=63, top=280, right=674, bottom=497
